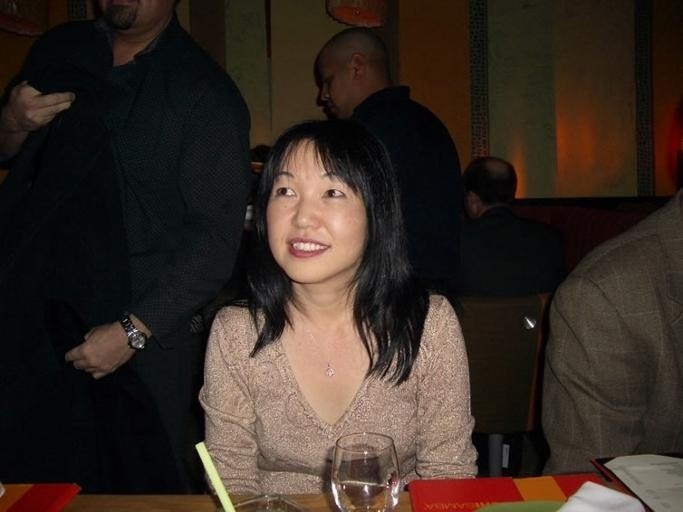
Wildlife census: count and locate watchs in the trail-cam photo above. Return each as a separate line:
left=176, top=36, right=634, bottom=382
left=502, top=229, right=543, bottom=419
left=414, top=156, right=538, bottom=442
left=118, top=313, right=147, bottom=352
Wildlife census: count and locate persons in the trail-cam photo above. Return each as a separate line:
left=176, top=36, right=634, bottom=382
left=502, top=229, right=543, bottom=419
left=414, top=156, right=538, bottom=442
left=312, top=25, right=467, bottom=333
left=538, top=190, right=683, bottom=472
left=459, top=155, right=573, bottom=298
left=196, top=117, right=480, bottom=494
left=0, top=2, right=256, bottom=495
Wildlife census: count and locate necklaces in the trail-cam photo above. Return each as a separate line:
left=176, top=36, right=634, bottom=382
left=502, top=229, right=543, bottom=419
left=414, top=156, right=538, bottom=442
left=295, top=320, right=364, bottom=380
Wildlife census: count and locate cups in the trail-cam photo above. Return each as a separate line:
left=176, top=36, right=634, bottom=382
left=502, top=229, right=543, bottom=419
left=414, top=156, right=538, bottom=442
left=331, top=433, right=400, bottom=511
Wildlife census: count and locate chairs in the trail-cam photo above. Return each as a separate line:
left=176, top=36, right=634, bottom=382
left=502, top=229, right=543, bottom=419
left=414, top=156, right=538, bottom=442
left=452, top=290, right=553, bottom=476
